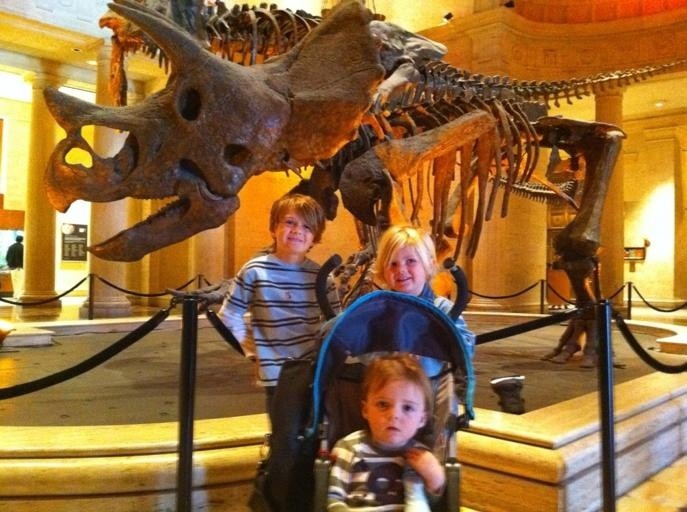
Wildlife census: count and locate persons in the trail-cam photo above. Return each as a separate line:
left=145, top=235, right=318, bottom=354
left=323, top=354, right=447, bottom=512
left=373, top=223, right=476, bottom=376
left=216, top=194, right=344, bottom=416
left=4, top=235, right=23, bottom=268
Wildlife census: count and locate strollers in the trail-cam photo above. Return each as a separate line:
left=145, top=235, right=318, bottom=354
left=305, top=254, right=476, bottom=511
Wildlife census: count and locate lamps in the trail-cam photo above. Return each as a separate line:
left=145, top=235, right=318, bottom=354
left=489, top=375, right=528, bottom=416
